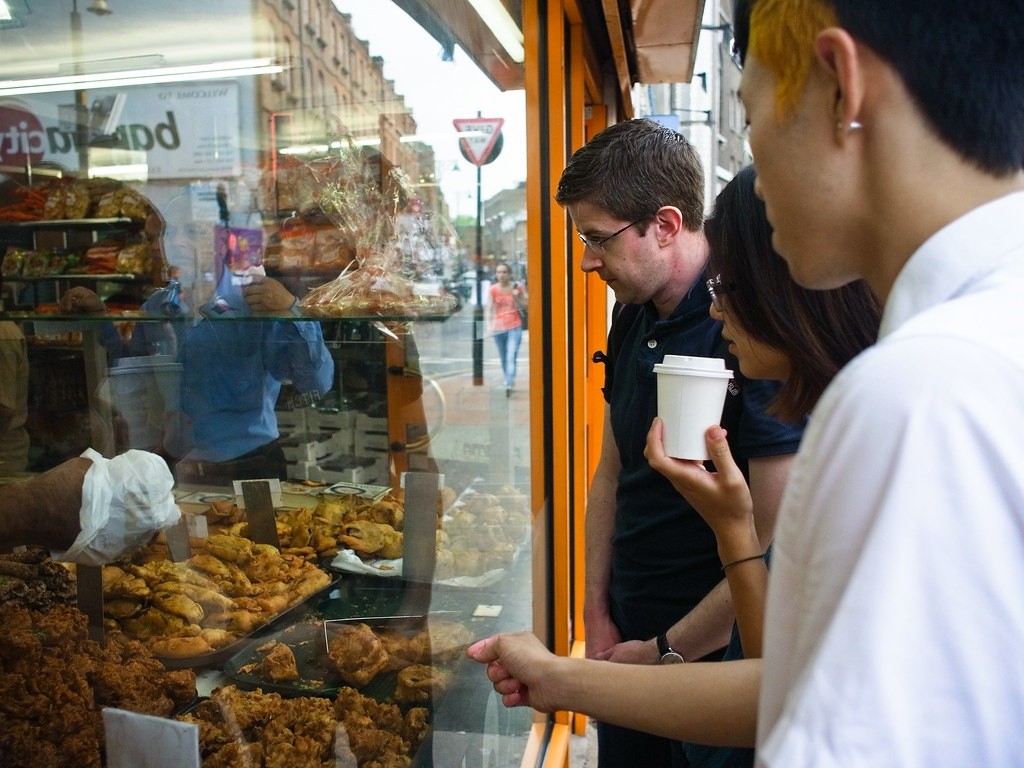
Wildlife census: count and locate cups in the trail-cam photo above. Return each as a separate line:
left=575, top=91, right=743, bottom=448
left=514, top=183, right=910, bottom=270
left=653, top=355, right=734, bottom=461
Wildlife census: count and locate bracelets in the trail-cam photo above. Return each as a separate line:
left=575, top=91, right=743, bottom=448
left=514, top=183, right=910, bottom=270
left=723, top=553, right=764, bottom=571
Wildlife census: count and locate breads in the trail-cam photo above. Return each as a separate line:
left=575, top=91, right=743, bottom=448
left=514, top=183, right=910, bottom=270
left=252, top=145, right=462, bottom=318
left=25, top=176, right=150, bottom=220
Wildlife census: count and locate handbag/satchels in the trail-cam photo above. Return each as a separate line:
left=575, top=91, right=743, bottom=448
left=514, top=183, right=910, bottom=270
left=516, top=307, right=528, bottom=331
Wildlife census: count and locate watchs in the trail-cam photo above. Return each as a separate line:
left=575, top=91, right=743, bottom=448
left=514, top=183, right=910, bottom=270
left=656, top=632, right=685, bottom=667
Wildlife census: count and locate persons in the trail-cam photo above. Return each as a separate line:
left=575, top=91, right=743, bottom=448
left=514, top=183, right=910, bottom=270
left=0, top=308, right=31, bottom=471
left=0, top=448, right=181, bottom=568
left=267, top=193, right=442, bottom=768
left=555, top=118, right=801, bottom=767
left=466, top=0, right=1024, bottom=768
left=57, top=184, right=334, bottom=488
left=642, top=160, right=888, bottom=768
left=488, top=262, right=529, bottom=399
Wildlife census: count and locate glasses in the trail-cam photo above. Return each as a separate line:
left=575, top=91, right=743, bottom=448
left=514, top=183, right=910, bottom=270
left=576, top=212, right=655, bottom=261
left=707, top=275, right=732, bottom=314
